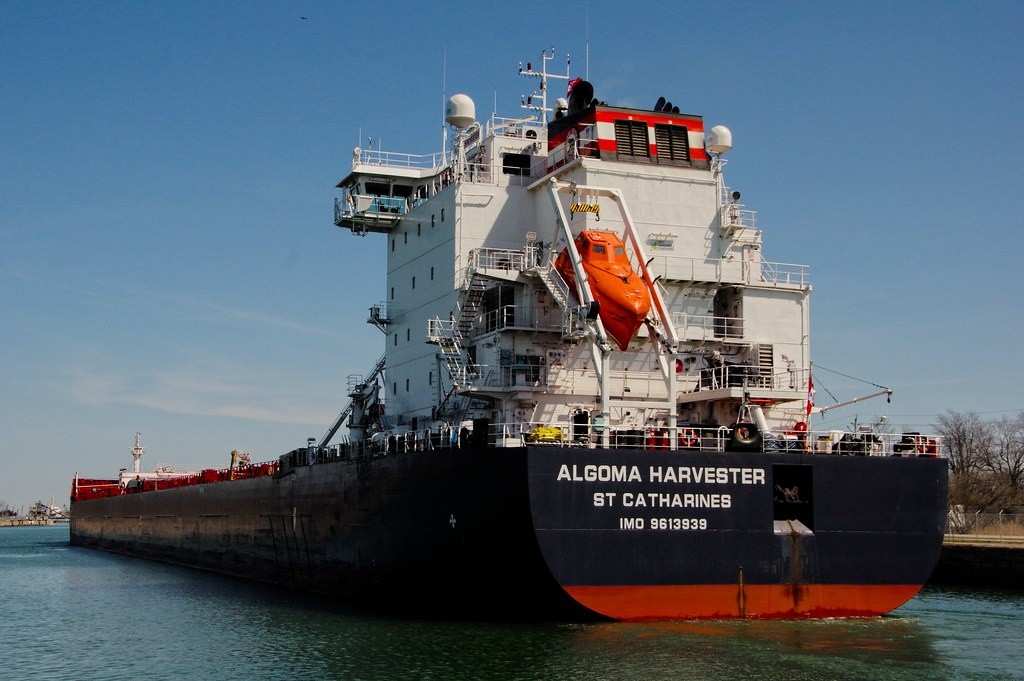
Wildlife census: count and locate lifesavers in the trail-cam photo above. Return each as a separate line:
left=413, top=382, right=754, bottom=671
left=680, top=427, right=698, bottom=445
left=916, top=437, right=931, bottom=452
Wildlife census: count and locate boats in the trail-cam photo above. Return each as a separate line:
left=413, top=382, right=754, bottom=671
left=67, top=46, right=954, bottom=623
left=554, top=228, right=651, bottom=353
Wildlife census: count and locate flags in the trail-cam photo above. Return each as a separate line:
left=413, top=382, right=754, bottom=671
left=807, top=377, right=816, bottom=415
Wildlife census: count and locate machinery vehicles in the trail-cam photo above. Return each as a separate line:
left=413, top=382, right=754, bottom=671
left=227, top=448, right=251, bottom=480
